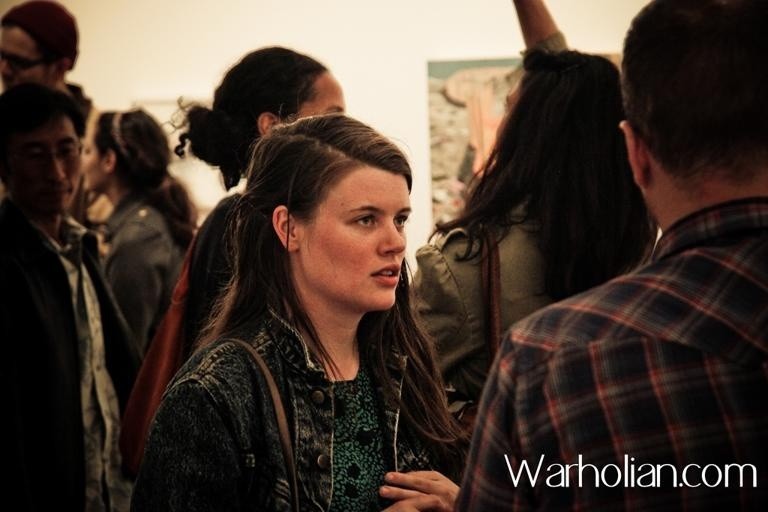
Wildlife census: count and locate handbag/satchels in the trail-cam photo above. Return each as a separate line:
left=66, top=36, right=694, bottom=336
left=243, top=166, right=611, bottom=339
left=118, top=273, right=187, bottom=473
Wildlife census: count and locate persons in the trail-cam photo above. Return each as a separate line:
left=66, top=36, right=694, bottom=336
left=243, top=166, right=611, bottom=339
left=0, top=1, right=766, bottom=510
